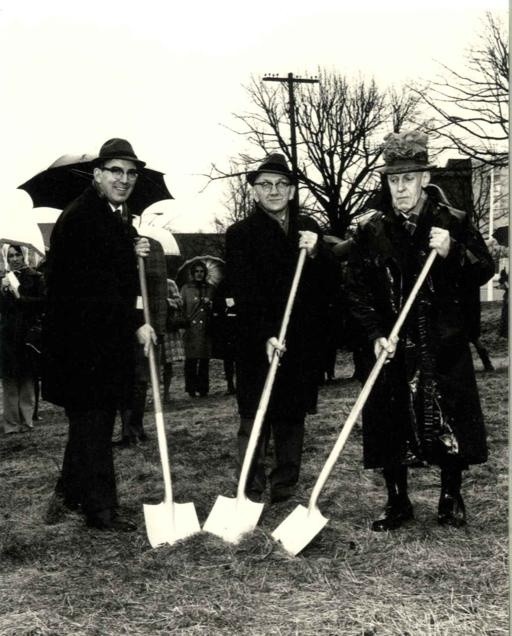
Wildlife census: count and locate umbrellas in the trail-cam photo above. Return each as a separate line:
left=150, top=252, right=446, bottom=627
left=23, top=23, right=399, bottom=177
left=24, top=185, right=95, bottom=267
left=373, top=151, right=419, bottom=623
left=138, top=227, right=180, bottom=256
left=16, top=154, right=174, bottom=215
left=0, top=223, right=45, bottom=257
left=492, top=225, right=508, bottom=247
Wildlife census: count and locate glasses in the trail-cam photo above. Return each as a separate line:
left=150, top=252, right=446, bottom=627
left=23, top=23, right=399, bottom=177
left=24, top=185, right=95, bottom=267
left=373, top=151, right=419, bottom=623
left=253, top=181, right=291, bottom=190
left=103, top=166, right=138, bottom=180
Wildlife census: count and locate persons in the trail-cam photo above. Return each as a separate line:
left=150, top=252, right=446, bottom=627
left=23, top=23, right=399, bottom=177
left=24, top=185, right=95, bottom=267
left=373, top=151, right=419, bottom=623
left=317, top=241, right=365, bottom=384
left=216, top=155, right=343, bottom=504
left=179, top=263, right=219, bottom=399
left=41, top=138, right=160, bottom=534
left=464, top=236, right=496, bottom=372
left=342, top=171, right=496, bottom=531
left=1, top=246, right=44, bottom=431
left=117, top=237, right=181, bottom=448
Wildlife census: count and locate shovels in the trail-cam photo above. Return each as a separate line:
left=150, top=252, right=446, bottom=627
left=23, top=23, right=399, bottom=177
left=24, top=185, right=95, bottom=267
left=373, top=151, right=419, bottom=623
left=270, top=249, right=436, bottom=558
left=139, top=256, right=201, bottom=549
left=200, top=248, right=315, bottom=546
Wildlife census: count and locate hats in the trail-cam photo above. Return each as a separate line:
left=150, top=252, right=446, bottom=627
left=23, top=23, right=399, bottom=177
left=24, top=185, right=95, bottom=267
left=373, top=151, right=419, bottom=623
left=246, top=154, right=300, bottom=189
left=377, top=130, right=436, bottom=175
left=92, top=138, right=146, bottom=169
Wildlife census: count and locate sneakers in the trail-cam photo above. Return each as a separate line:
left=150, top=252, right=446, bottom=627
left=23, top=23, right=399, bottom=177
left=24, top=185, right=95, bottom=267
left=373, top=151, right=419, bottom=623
left=87, top=512, right=136, bottom=532
left=247, top=480, right=299, bottom=502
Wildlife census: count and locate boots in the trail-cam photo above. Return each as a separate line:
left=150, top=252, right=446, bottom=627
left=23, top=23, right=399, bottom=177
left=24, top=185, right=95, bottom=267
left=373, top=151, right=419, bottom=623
left=374, top=467, right=413, bottom=530
left=437, top=469, right=465, bottom=526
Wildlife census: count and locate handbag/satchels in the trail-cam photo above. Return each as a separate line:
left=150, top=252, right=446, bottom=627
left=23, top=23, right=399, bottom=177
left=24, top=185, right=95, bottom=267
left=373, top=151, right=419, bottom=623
left=9, top=266, right=43, bottom=296
left=167, top=309, right=191, bottom=329
